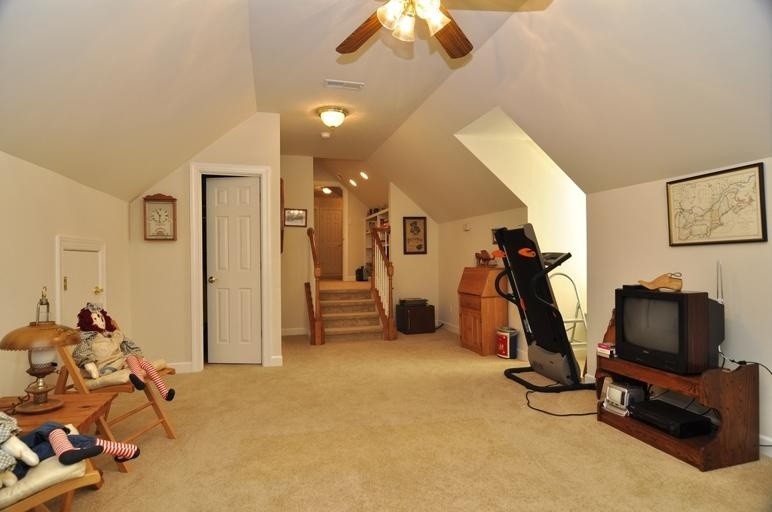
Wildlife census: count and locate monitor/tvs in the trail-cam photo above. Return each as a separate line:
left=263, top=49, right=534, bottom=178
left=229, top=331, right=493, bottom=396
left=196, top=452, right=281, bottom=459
left=615, top=288, right=725, bottom=376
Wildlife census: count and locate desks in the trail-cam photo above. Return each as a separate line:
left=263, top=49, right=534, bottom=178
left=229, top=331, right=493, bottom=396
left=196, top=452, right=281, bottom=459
left=2, top=393, right=117, bottom=490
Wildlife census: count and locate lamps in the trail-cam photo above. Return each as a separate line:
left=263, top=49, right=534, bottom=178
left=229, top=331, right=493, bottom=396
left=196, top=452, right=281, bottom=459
left=374, top=0, right=451, bottom=44
left=313, top=105, right=351, bottom=128
left=1, top=285, right=83, bottom=414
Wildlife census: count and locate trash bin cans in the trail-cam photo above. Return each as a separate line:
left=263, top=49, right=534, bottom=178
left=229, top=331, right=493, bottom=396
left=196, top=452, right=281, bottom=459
left=496, top=327, right=518, bottom=359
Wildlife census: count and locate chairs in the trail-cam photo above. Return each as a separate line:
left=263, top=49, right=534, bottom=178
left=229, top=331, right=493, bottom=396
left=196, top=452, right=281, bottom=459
left=0, top=434, right=103, bottom=512
left=53, top=318, right=175, bottom=475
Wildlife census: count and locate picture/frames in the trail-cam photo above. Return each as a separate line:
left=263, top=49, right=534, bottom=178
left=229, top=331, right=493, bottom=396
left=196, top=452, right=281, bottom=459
left=284, top=208, right=308, bottom=228
left=665, top=161, right=768, bottom=247
left=402, top=216, right=428, bottom=255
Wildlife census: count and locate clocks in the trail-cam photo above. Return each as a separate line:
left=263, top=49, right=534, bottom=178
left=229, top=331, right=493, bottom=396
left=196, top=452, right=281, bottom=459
left=143, top=193, right=178, bottom=241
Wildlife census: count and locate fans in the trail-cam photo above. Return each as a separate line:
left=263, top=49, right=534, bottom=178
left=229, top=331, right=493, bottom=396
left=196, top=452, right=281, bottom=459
left=336, top=0, right=472, bottom=60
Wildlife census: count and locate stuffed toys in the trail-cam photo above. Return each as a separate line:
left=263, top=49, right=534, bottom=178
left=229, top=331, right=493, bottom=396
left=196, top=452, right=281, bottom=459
left=10, top=420, right=142, bottom=482
left=0, top=409, right=40, bottom=488
left=72, top=301, right=175, bottom=401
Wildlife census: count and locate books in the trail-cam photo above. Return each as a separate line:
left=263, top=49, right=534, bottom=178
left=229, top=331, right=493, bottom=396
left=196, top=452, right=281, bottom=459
left=597, top=352, right=617, bottom=359
left=597, top=342, right=617, bottom=349
left=596, top=347, right=616, bottom=355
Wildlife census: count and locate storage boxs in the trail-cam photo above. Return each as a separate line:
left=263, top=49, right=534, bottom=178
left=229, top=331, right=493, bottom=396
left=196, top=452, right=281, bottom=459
left=394, top=301, right=437, bottom=334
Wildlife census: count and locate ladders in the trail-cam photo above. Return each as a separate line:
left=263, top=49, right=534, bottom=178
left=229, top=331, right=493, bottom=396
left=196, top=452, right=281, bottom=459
left=548, top=273, right=586, bottom=352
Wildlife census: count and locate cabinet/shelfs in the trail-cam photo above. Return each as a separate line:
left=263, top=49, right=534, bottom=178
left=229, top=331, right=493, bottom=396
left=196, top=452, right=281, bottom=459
left=596, top=345, right=759, bottom=470
left=457, top=263, right=512, bottom=356
left=362, top=208, right=390, bottom=264
